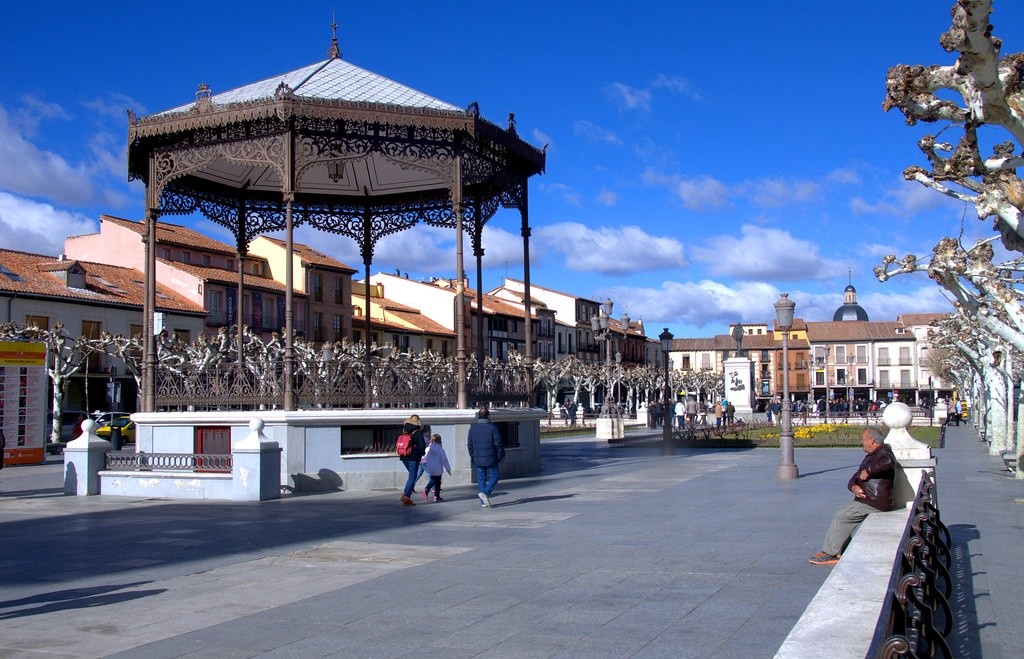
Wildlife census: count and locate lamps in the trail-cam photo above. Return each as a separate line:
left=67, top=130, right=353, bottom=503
left=326, top=151, right=347, bottom=182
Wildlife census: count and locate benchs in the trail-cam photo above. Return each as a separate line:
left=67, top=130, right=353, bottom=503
left=770, top=466, right=953, bottom=659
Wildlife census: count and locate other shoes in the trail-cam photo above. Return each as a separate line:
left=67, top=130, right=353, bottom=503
left=422, top=490, right=428, bottom=500
left=482, top=505, right=487, bottom=507
left=808, top=552, right=842, bottom=564
left=400, top=493, right=414, bottom=506
left=478, top=493, right=491, bottom=506
left=433, top=496, right=443, bottom=501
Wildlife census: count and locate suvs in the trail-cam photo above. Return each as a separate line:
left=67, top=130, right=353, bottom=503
left=48, top=410, right=135, bottom=445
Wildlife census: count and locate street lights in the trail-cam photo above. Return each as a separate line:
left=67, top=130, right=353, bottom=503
left=615, top=351, right=624, bottom=414
left=773, top=293, right=799, bottom=479
left=323, top=341, right=334, bottom=409
left=590, top=298, right=631, bottom=440
left=658, top=328, right=674, bottom=454
left=802, top=352, right=820, bottom=406
left=848, top=352, right=855, bottom=416
left=823, top=344, right=831, bottom=417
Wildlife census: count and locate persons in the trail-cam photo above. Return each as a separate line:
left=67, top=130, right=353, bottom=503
left=468, top=408, right=505, bottom=508
left=407, top=426, right=446, bottom=492
left=0, top=418, right=6, bottom=470
left=71, top=413, right=87, bottom=439
left=650, top=399, right=735, bottom=429
left=809, top=426, right=897, bottom=564
left=763, top=396, right=962, bottom=426
left=422, top=434, right=451, bottom=502
left=396, top=414, right=426, bottom=506
left=565, top=401, right=577, bottom=425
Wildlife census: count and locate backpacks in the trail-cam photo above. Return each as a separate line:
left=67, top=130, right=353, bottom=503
left=396, top=428, right=418, bottom=456
